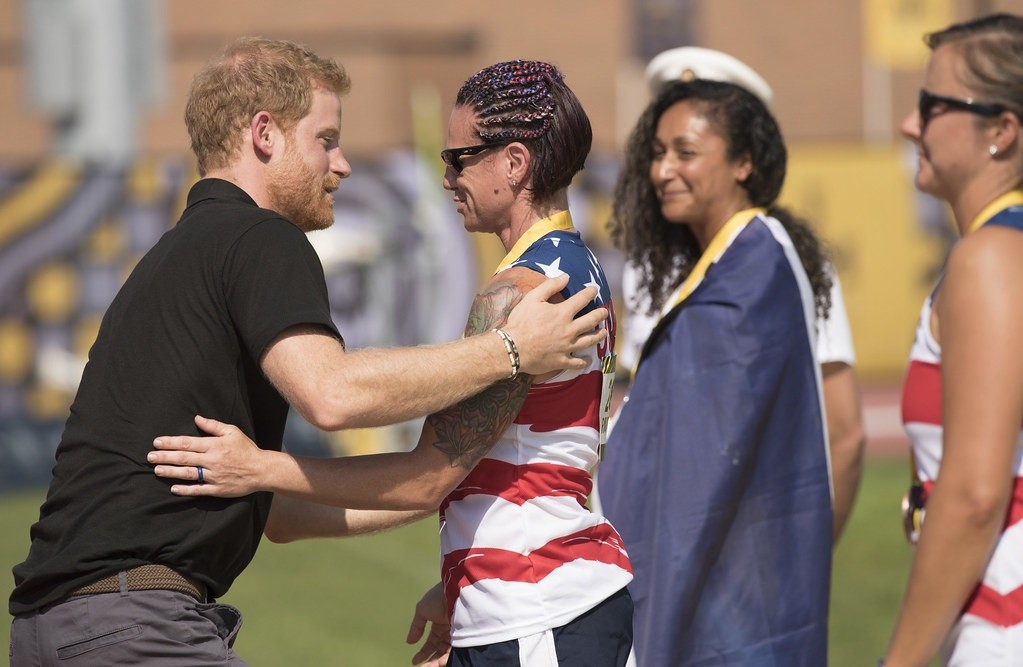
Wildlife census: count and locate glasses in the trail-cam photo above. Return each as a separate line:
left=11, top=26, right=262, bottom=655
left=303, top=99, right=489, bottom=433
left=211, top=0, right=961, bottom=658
left=917, top=88, right=1023, bottom=132
left=441, top=140, right=511, bottom=176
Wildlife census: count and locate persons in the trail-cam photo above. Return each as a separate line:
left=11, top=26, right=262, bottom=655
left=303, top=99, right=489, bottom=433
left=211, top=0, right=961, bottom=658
left=148, top=60, right=638, bottom=667
left=9, top=37, right=611, bottom=666
left=876, top=12, right=1023, bottom=667
left=596, top=47, right=868, bottom=667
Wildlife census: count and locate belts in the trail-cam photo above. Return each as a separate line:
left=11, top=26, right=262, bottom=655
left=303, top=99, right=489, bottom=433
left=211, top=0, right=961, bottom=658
left=68, top=565, right=206, bottom=607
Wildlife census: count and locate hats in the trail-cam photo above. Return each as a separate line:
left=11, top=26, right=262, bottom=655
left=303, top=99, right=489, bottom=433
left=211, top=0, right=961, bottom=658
left=644, top=42, right=774, bottom=111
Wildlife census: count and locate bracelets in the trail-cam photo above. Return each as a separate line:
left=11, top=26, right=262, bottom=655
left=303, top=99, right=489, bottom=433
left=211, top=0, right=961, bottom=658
left=492, top=328, right=521, bottom=382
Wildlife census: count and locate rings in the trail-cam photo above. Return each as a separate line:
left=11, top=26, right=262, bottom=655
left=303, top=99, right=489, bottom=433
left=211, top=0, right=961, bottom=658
left=198, top=467, right=204, bottom=483
